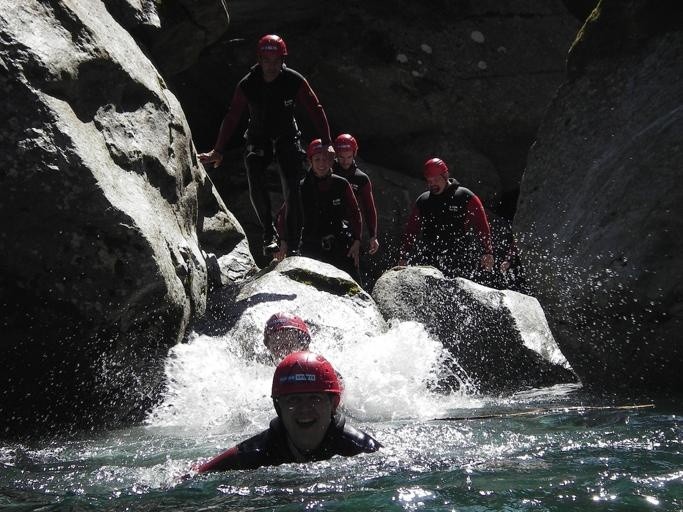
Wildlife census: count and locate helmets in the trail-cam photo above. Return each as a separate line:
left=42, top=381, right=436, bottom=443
left=264, top=312, right=312, bottom=345
left=333, top=133, right=359, bottom=153
left=424, top=158, right=448, bottom=178
left=272, top=350, right=341, bottom=398
left=307, top=138, right=336, bottom=158
left=256, top=34, right=287, bottom=58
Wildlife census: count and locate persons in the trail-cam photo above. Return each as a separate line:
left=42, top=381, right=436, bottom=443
left=330, top=131, right=380, bottom=256
left=273, top=136, right=365, bottom=289
left=398, top=157, right=496, bottom=279
left=193, top=349, right=384, bottom=475
left=195, top=34, right=332, bottom=254
left=246, top=310, right=346, bottom=395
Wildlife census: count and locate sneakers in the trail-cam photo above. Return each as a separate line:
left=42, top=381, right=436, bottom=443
left=262, top=228, right=280, bottom=257
